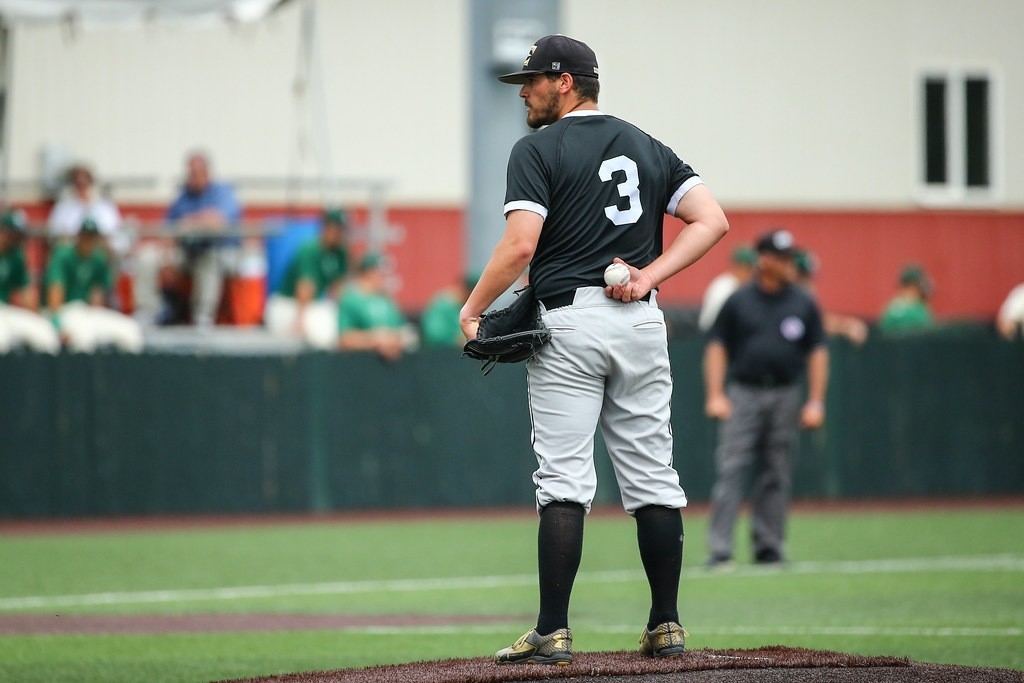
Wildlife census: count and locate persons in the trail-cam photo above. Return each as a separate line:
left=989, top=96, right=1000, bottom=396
left=700, top=246, right=869, bottom=346
left=880, top=267, right=936, bottom=335
left=0, top=154, right=533, bottom=362
left=705, top=230, right=831, bottom=568
left=996, top=282, right=1024, bottom=340
left=458, top=33, right=730, bottom=666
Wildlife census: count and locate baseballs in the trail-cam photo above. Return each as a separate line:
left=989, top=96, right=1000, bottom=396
left=602, top=262, right=631, bottom=288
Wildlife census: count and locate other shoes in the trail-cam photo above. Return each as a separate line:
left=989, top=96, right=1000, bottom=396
left=754, top=553, right=784, bottom=570
left=707, top=555, right=735, bottom=570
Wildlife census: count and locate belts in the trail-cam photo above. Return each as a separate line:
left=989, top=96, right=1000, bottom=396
left=544, top=286, right=651, bottom=310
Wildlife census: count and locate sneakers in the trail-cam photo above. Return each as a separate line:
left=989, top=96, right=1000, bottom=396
left=493, top=627, right=574, bottom=665
left=638, top=622, right=688, bottom=658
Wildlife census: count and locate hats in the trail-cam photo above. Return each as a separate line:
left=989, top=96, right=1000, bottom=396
left=361, top=254, right=383, bottom=269
left=1, top=207, right=31, bottom=242
left=325, top=207, right=346, bottom=225
left=901, top=267, right=920, bottom=283
left=756, top=229, right=803, bottom=258
left=735, top=250, right=754, bottom=261
left=465, top=270, right=483, bottom=290
left=497, top=34, right=598, bottom=85
left=79, top=220, right=101, bottom=235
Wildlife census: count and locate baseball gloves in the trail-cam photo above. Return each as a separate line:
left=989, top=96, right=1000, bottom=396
left=461, top=285, right=553, bottom=365
